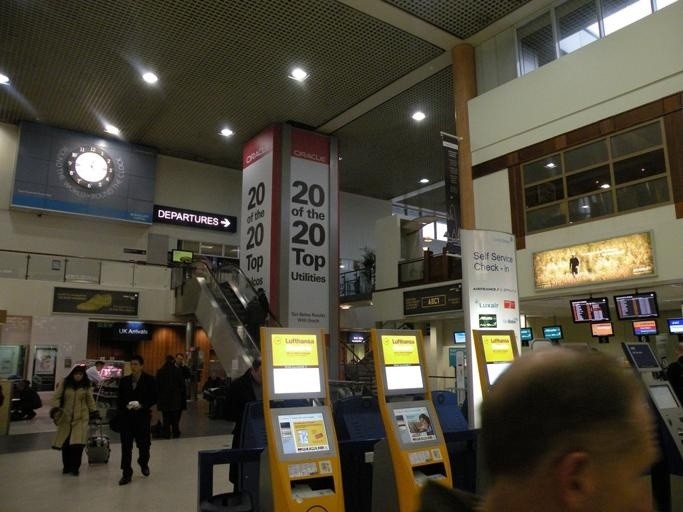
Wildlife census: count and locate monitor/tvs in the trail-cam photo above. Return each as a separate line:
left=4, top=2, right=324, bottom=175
left=454, top=332, right=466, bottom=344
left=632, top=320, right=658, bottom=336
left=590, top=322, right=614, bottom=337
left=521, top=327, right=533, bottom=341
left=666, top=318, right=683, bottom=335
left=542, top=325, right=563, bottom=340
left=570, top=297, right=611, bottom=323
left=614, top=292, right=659, bottom=320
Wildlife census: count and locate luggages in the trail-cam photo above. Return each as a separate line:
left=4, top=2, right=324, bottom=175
left=83, top=415, right=112, bottom=466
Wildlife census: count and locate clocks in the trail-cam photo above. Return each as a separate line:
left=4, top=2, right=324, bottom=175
left=63, top=143, right=116, bottom=192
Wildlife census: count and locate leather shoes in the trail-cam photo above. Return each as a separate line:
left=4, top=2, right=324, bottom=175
left=117, top=469, right=134, bottom=486
left=74, top=291, right=114, bottom=313
left=136, top=456, right=152, bottom=476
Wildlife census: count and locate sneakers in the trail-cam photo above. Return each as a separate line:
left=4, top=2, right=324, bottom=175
left=62, top=466, right=80, bottom=476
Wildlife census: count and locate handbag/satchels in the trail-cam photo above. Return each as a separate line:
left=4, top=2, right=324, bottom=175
left=48, top=377, right=69, bottom=426
left=106, top=410, right=121, bottom=433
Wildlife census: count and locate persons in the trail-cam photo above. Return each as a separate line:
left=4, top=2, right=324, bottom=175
left=19, top=380, right=42, bottom=420
left=257, top=288, right=269, bottom=328
left=86, top=360, right=111, bottom=383
left=245, top=295, right=261, bottom=347
left=156, top=350, right=204, bottom=439
left=116, top=355, right=158, bottom=485
left=570, top=254, right=579, bottom=275
left=48, top=365, right=99, bottom=476
left=416, top=344, right=660, bottom=511
